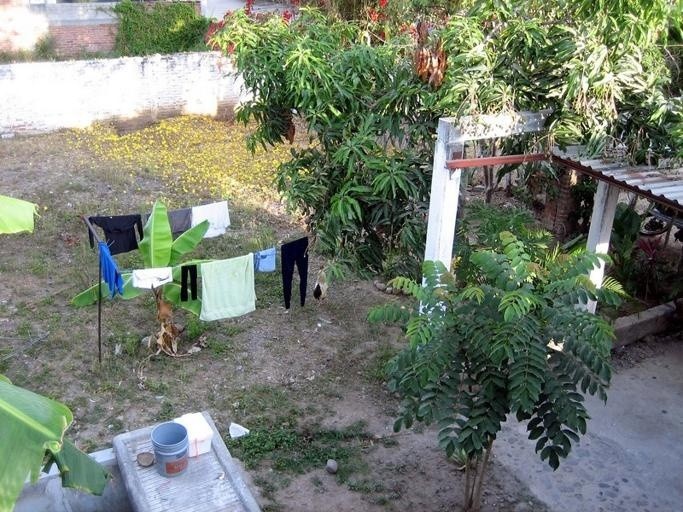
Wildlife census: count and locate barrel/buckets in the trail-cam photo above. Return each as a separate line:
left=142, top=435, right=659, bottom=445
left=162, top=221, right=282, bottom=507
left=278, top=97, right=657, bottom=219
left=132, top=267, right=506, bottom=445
left=151, top=421, right=189, bottom=477
